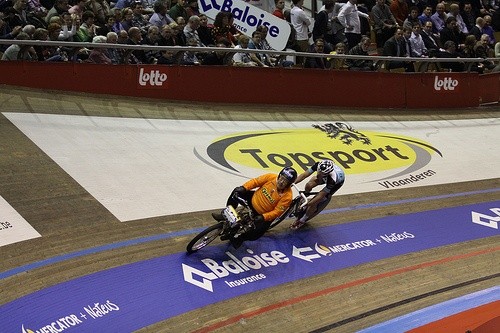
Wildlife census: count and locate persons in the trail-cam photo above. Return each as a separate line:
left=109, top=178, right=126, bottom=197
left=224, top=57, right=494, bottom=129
left=212, top=165, right=297, bottom=248
left=0, top=0, right=500, bottom=73
left=291, top=160, right=345, bottom=230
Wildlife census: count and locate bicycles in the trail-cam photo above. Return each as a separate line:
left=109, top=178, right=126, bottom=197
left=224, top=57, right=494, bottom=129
left=186, top=195, right=255, bottom=253
left=267, top=182, right=331, bottom=231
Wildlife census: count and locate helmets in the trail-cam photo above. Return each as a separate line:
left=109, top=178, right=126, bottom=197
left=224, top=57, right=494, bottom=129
left=278, top=167, right=297, bottom=187
left=317, top=161, right=333, bottom=175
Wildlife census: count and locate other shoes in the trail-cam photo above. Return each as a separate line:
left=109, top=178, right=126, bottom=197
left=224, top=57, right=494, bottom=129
left=212, top=214, right=225, bottom=222
left=291, top=220, right=302, bottom=230
